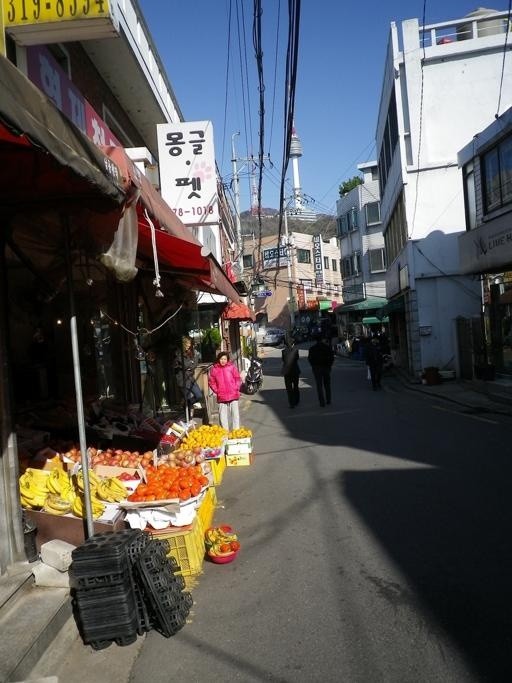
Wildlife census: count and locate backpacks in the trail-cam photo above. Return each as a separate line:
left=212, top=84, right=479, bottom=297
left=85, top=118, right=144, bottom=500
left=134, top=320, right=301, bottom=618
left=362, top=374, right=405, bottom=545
left=282, top=349, right=297, bottom=376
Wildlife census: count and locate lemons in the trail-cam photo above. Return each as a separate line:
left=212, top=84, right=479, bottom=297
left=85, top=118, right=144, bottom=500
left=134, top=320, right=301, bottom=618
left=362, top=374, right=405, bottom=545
left=180, top=424, right=251, bottom=450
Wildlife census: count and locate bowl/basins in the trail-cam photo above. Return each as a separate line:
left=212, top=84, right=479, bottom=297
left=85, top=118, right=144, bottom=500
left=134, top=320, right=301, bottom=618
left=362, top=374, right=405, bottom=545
left=206, top=550, right=236, bottom=564
left=205, top=533, right=239, bottom=549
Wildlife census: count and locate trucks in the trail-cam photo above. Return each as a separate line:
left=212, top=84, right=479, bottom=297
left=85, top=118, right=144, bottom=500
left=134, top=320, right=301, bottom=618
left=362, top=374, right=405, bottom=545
left=310, top=318, right=337, bottom=338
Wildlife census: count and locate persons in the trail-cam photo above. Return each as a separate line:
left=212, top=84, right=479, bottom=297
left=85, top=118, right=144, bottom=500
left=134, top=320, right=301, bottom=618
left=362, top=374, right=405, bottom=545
left=344, top=330, right=391, bottom=355
left=307, top=335, right=334, bottom=407
left=173, top=336, right=203, bottom=405
left=365, top=339, right=384, bottom=392
left=282, top=338, right=301, bottom=409
left=208, top=352, right=242, bottom=432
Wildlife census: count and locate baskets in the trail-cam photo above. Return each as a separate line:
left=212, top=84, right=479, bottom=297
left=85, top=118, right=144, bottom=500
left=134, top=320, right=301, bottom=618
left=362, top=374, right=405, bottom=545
left=148, top=452, right=226, bottom=578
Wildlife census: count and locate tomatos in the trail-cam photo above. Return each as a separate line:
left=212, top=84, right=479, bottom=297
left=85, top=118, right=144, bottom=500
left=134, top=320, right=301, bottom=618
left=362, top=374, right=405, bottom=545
left=128, top=464, right=209, bottom=501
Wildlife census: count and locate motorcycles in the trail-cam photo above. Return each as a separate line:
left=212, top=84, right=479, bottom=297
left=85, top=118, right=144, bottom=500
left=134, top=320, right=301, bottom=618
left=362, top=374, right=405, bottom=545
left=246, top=358, right=263, bottom=395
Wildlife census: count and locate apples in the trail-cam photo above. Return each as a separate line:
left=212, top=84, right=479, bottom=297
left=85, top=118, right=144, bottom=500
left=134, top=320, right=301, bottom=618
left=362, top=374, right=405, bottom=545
left=220, top=526, right=232, bottom=533
left=65, top=446, right=153, bottom=469
left=221, top=541, right=240, bottom=552
left=165, top=446, right=204, bottom=467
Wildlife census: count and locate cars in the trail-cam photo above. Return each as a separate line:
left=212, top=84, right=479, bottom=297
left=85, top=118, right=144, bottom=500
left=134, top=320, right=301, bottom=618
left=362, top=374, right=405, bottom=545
left=263, top=329, right=285, bottom=346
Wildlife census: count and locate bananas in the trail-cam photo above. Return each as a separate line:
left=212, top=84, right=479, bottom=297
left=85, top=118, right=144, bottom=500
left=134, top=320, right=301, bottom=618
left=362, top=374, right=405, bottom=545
left=19, top=466, right=128, bottom=522
